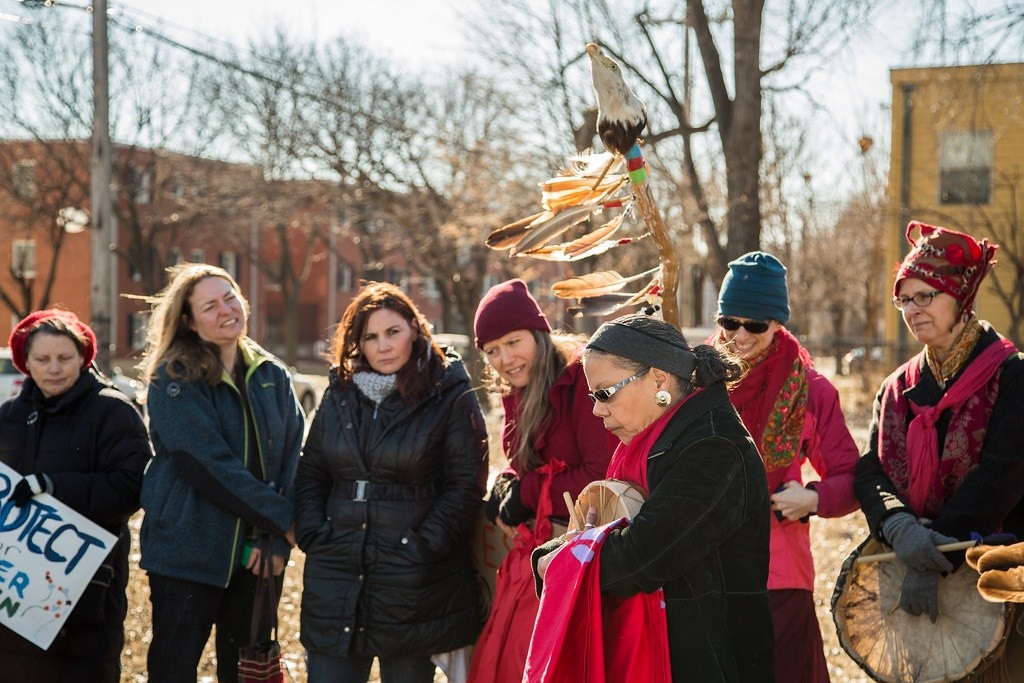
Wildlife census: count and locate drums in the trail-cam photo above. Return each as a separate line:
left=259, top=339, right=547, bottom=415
left=830, top=518, right=1015, bottom=683
left=567, top=478, right=650, bottom=533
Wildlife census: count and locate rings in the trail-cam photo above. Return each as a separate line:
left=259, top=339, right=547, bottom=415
left=584, top=523, right=596, bottom=529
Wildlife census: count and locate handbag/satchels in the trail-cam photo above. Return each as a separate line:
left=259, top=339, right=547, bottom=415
left=237, top=533, right=284, bottom=683
left=476, top=472, right=571, bottom=607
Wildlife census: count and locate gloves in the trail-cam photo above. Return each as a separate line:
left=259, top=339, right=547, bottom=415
left=10, top=473, right=54, bottom=508
left=900, top=566, right=938, bottom=623
left=881, top=512, right=959, bottom=575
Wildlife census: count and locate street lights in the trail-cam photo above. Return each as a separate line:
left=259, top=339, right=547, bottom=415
left=20, top=0, right=113, bottom=382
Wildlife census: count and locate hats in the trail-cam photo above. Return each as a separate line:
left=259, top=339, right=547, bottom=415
left=717, top=251, right=792, bottom=326
left=894, top=220, right=999, bottom=333
left=474, top=278, right=551, bottom=351
left=9, top=310, right=97, bottom=377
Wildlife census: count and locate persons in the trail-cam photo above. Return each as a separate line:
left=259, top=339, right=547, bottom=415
left=538, top=313, right=778, bottom=683
left=704, top=252, right=859, bottom=683
left=854, top=219, right=1024, bottom=683
left=0, top=309, right=153, bottom=683
left=481, top=280, right=621, bottom=538
left=295, top=278, right=488, bottom=683
left=137, top=261, right=307, bottom=683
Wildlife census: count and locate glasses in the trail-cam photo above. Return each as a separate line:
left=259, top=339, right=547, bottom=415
left=588, top=366, right=650, bottom=404
left=717, top=314, right=773, bottom=334
left=892, top=289, right=944, bottom=311
